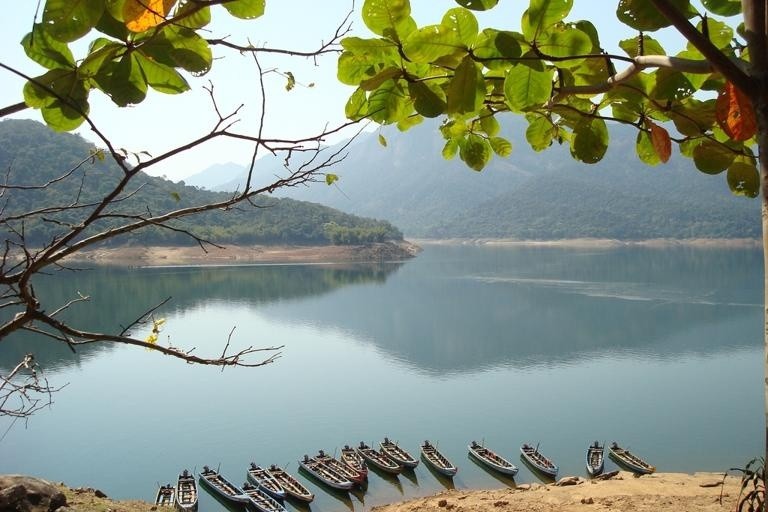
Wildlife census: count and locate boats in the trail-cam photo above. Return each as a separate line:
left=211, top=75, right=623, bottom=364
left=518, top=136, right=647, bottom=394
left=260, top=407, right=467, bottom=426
left=518, top=442, right=561, bottom=478
left=151, top=483, right=175, bottom=511
left=585, top=439, right=605, bottom=475
left=465, top=439, right=518, bottom=477
left=172, top=468, right=200, bottom=512
left=607, top=441, right=656, bottom=474
left=198, top=479, right=249, bottom=512
left=239, top=438, right=458, bottom=512
left=519, top=455, right=556, bottom=485
left=463, top=451, right=517, bottom=490
left=195, top=465, right=250, bottom=506
